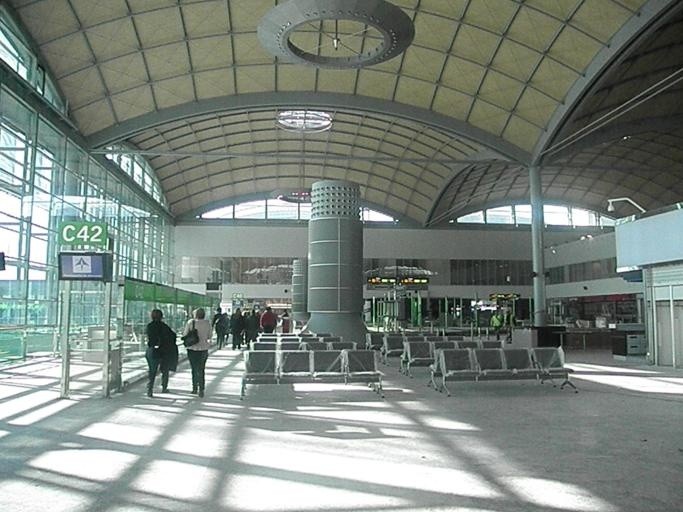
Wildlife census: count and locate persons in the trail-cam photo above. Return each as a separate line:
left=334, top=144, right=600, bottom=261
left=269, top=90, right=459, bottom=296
left=143, top=310, right=177, bottom=397
left=210, top=306, right=290, bottom=350
left=489, top=310, right=503, bottom=328
left=182, top=307, right=213, bottom=398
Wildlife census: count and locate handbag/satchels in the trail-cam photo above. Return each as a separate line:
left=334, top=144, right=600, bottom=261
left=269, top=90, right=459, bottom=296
left=183, top=329, right=199, bottom=347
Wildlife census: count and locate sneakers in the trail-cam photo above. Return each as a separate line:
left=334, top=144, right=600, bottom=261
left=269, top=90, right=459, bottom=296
left=162, top=390, right=169, bottom=393
left=199, top=386, right=204, bottom=397
left=148, top=390, right=152, bottom=397
left=192, top=390, right=197, bottom=394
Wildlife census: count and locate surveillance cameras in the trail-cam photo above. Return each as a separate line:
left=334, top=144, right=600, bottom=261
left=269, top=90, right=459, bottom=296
left=607, top=202, right=615, bottom=213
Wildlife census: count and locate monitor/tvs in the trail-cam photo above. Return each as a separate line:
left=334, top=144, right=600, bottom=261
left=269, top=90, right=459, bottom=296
left=58, top=252, right=105, bottom=280
